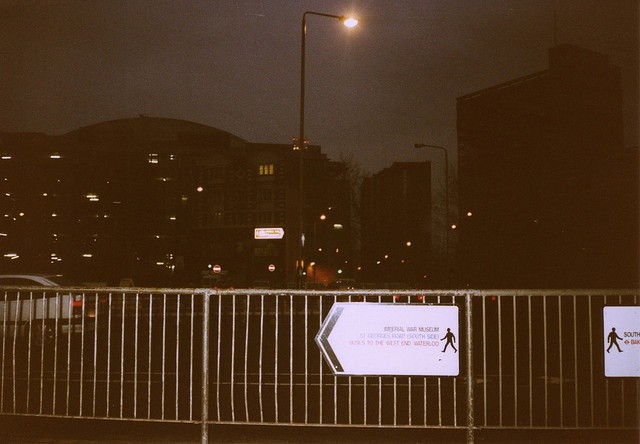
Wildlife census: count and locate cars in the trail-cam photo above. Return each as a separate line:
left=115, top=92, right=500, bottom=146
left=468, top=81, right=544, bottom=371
left=0, top=273, right=89, bottom=347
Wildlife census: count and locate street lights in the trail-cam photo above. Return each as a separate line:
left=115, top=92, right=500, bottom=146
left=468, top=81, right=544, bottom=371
left=297, top=9, right=360, bottom=285
left=414, top=141, right=452, bottom=288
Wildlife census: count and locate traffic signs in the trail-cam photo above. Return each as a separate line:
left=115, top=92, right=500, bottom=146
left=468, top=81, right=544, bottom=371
left=254, top=228, right=285, bottom=239
left=314, top=301, right=461, bottom=377
left=603, top=305, right=640, bottom=379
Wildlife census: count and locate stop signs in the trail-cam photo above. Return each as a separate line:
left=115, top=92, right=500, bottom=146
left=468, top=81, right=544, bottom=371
left=213, top=265, right=221, bottom=274
left=268, top=265, right=275, bottom=272
